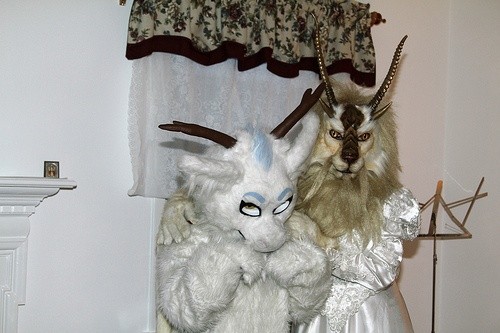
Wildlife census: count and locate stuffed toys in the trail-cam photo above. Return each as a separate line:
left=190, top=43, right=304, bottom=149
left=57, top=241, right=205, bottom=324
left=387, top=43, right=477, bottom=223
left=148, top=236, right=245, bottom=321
left=156, top=12, right=424, bottom=333
left=156, top=82, right=333, bottom=333
